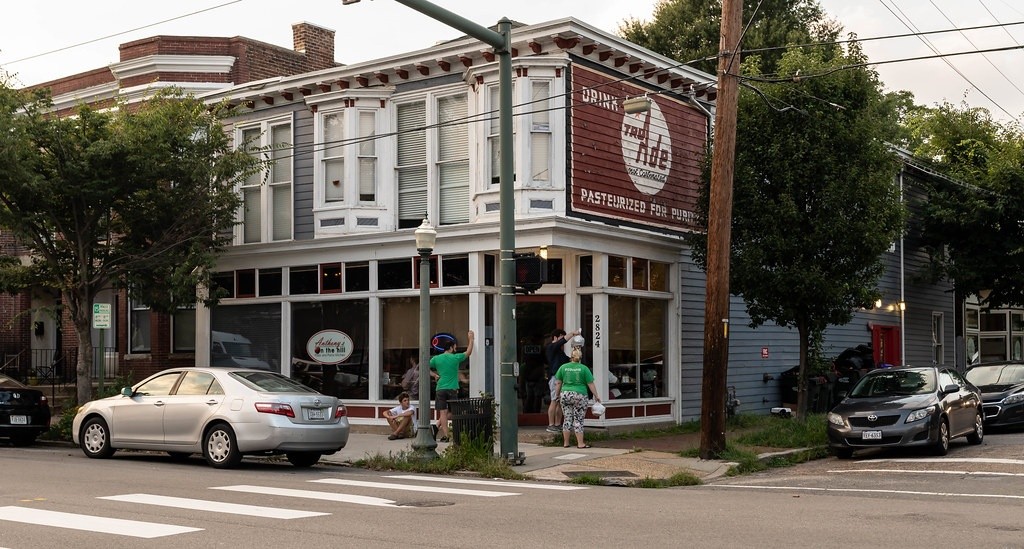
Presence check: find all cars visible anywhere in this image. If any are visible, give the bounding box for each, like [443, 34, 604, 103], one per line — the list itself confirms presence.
[211, 330, 468, 407]
[959, 359, 1024, 434]
[826, 362, 986, 460]
[0, 373, 52, 448]
[72, 366, 351, 469]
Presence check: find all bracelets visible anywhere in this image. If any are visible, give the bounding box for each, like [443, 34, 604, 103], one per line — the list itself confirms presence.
[573, 333, 574, 336]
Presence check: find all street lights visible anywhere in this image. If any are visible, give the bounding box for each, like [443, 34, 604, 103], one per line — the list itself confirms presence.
[407, 210, 441, 461]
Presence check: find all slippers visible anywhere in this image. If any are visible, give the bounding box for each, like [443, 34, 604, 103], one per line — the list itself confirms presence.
[577, 444, 591, 448]
[564, 445, 571, 448]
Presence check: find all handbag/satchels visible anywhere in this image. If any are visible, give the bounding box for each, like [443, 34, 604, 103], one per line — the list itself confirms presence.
[591, 401, 606, 417]
[571, 328, 584, 348]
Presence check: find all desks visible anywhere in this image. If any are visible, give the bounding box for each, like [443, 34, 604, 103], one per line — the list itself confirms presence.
[609, 380, 655, 399]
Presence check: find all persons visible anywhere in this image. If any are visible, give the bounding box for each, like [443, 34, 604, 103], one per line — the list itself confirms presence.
[541, 329, 581, 432]
[553, 350, 603, 449]
[523, 365, 549, 399]
[382, 393, 417, 440]
[395, 355, 420, 399]
[429, 331, 474, 442]
[609, 362, 644, 399]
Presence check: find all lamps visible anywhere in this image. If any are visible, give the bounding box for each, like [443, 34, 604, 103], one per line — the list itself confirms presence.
[896, 300, 906, 310]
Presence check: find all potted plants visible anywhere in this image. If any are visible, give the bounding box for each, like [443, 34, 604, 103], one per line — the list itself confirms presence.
[28, 369, 38, 386]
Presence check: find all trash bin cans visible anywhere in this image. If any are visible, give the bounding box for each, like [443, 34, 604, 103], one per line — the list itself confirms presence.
[446, 397, 495, 455]
[781, 367, 868, 415]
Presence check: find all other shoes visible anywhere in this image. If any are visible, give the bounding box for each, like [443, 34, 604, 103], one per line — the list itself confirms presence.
[388, 433, 398, 440]
[555, 424, 563, 432]
[397, 432, 404, 439]
[440, 436, 450, 443]
[546, 424, 559, 433]
[431, 424, 439, 441]
